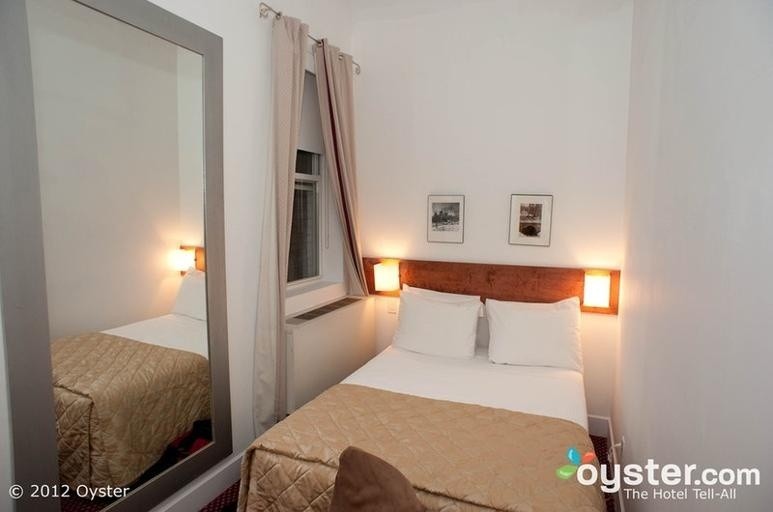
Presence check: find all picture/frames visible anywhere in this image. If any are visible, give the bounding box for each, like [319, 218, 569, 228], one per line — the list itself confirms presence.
[427, 194, 464, 245]
[506, 193, 553, 246]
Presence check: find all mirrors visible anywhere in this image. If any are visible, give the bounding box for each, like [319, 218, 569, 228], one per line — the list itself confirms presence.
[0, 1, 233, 510]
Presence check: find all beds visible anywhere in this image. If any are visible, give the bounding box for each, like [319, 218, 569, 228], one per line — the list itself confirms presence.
[237, 343, 609, 512]
[48, 313, 214, 498]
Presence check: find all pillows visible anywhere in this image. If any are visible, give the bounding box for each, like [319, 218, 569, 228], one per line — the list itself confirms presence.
[172, 269, 207, 320]
[393, 283, 583, 369]
